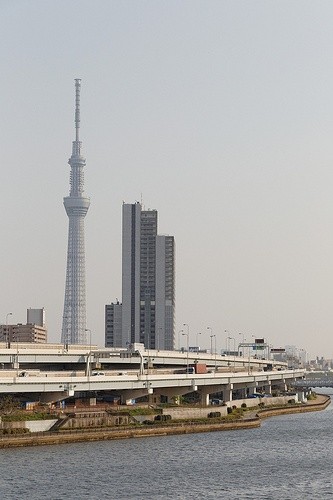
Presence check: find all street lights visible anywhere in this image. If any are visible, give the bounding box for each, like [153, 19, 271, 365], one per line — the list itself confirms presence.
[183, 333, 189, 351]
[229, 337, 236, 367]
[183, 323, 190, 373]
[141, 332, 150, 374]
[238, 332, 245, 366]
[85, 328, 91, 354]
[196, 333, 202, 353]
[6, 312, 12, 349]
[210, 336, 216, 371]
[207, 327, 213, 354]
